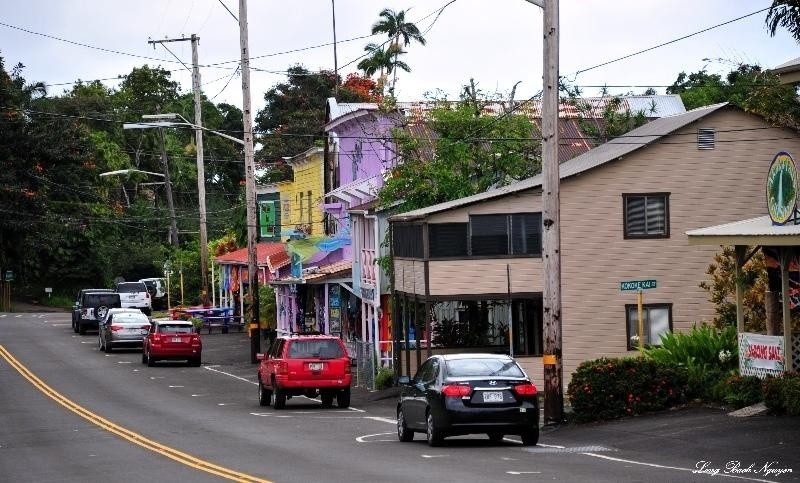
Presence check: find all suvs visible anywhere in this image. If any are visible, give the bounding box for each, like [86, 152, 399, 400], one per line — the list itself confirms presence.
[69, 273, 178, 353]
[252, 329, 355, 411]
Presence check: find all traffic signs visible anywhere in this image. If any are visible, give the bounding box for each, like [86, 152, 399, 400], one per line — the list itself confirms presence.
[620, 281, 658, 292]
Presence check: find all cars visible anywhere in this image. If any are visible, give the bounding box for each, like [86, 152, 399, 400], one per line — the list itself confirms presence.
[394, 350, 542, 447]
[140, 317, 202, 368]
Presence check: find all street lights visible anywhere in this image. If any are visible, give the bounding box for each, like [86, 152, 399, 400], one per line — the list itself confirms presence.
[119, 120, 264, 343]
[95, 168, 181, 249]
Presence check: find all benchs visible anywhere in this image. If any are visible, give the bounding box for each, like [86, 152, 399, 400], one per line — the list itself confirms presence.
[176, 303, 246, 335]
